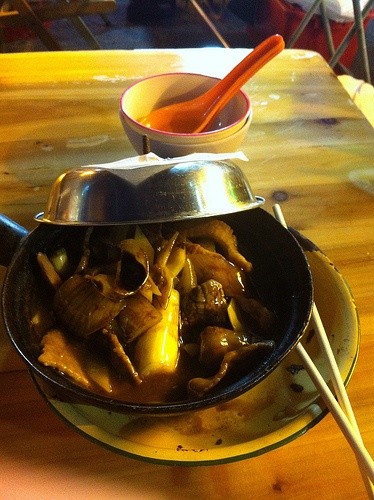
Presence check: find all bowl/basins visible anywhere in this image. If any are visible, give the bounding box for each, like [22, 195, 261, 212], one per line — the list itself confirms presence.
[34, 159, 266, 226]
[120, 73, 253, 160]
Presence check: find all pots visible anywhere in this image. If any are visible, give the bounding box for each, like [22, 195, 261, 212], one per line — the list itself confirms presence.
[0, 207, 313, 412]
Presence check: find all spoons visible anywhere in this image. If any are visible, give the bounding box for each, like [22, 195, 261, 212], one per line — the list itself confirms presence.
[147, 35, 284, 134]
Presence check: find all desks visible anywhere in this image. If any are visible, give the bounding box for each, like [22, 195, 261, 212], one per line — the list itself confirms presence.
[0, 48, 374, 500]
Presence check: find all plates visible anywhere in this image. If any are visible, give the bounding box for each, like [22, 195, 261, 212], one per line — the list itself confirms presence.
[29, 225, 361, 466]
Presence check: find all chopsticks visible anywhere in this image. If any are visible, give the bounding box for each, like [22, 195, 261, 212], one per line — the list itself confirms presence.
[272, 203, 374, 500]
